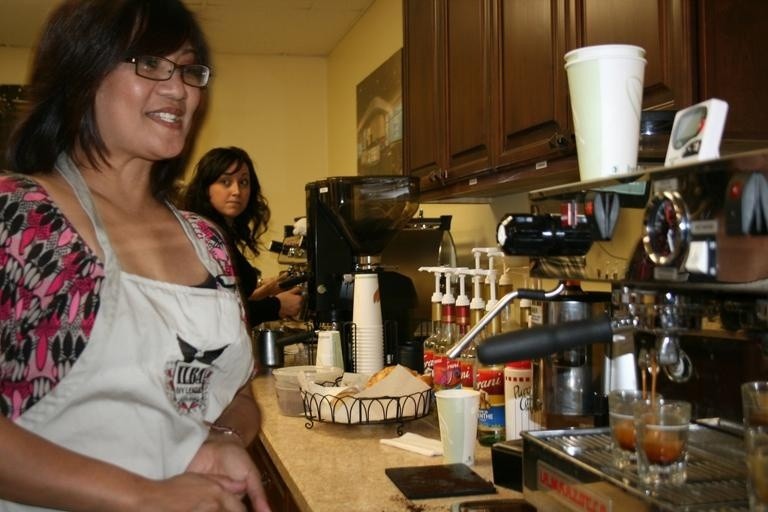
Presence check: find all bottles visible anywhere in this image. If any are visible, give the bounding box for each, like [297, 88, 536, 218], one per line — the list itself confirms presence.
[548, 275, 594, 430]
[416, 246, 542, 442]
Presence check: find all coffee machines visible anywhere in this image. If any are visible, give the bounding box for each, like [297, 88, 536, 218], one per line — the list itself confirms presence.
[316, 172, 418, 382]
[267, 214, 454, 344]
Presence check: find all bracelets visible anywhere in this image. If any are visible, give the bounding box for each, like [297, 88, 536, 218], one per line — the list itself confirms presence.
[203, 420, 244, 444]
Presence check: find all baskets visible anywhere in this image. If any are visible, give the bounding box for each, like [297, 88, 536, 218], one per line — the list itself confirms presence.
[300, 376, 433, 436]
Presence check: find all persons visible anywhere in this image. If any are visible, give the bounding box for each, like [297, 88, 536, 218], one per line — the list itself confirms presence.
[0, 0, 273, 512]
[179, 147, 304, 328]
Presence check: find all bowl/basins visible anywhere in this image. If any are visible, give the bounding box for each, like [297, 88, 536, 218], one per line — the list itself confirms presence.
[271, 364, 325, 417]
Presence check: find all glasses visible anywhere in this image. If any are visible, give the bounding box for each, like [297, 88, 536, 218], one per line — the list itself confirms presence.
[116, 49, 213, 89]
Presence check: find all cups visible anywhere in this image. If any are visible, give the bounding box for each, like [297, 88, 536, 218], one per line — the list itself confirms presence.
[352, 274, 386, 377]
[629, 396, 692, 485]
[562, 44, 645, 183]
[606, 387, 662, 469]
[435, 387, 482, 468]
[741, 379, 768, 512]
[314, 330, 345, 373]
[611, 287, 701, 330]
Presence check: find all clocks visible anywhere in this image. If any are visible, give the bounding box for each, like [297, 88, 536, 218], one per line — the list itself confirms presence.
[663, 97, 729, 168]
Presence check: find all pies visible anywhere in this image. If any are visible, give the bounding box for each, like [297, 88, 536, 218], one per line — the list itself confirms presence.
[329, 363, 435, 412]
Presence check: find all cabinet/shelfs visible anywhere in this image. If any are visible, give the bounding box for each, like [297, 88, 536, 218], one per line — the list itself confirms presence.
[402, 0, 767, 205]
